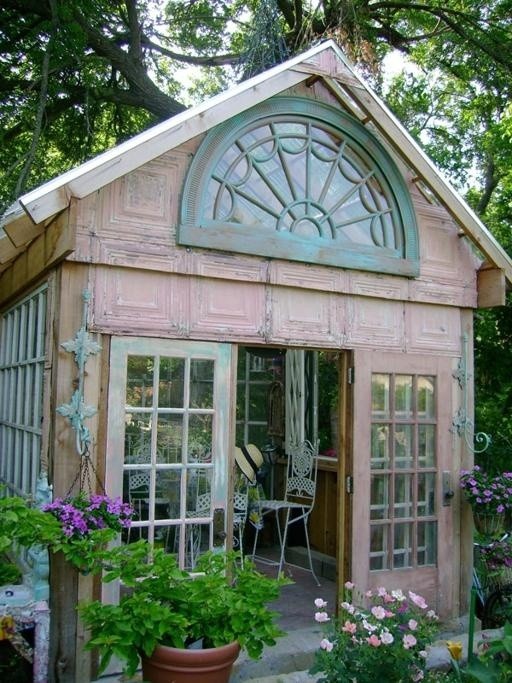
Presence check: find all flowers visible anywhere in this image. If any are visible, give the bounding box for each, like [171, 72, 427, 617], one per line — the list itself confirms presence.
[458, 465, 512, 513]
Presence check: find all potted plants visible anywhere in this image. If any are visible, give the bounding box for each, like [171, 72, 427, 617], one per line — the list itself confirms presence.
[75, 538, 296, 683]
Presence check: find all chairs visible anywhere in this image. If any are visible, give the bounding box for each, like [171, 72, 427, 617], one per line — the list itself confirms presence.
[128, 434, 322, 587]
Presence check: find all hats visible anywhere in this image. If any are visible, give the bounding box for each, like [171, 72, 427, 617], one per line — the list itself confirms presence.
[235, 444, 263, 484]
